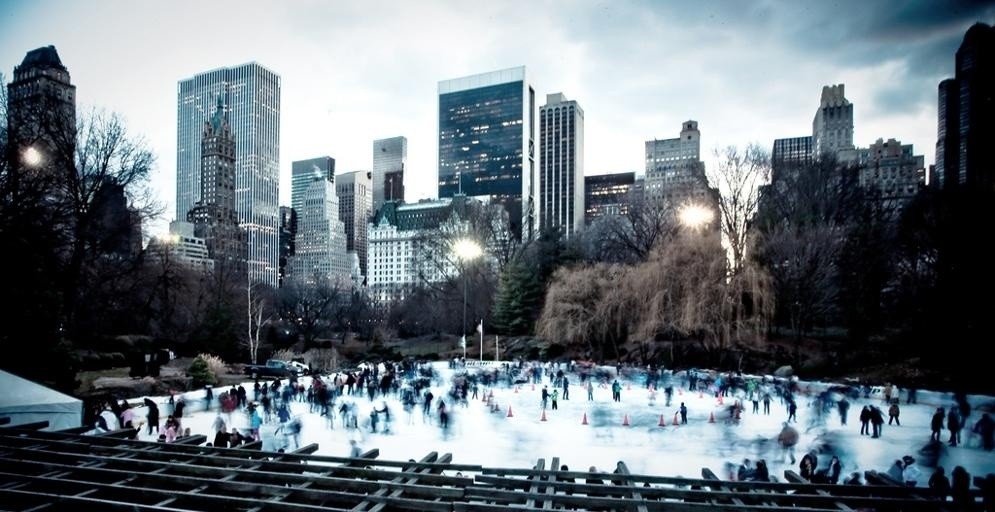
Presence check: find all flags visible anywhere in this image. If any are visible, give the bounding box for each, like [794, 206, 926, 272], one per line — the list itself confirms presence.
[458, 336, 466, 348]
[476, 324, 481, 332]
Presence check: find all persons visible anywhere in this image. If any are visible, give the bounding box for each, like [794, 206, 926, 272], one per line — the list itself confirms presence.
[718, 371, 995, 488]
[473, 347, 742, 427]
[101, 355, 474, 462]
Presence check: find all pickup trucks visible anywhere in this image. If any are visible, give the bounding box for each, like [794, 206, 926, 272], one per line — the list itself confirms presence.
[243, 359, 303, 380]
[286, 361, 309, 375]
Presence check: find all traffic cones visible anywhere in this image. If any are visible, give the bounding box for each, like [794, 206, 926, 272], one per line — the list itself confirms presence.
[482, 382, 536, 418]
[659, 414, 665, 426]
[698, 389, 743, 424]
[582, 412, 588, 424]
[672, 415, 679, 425]
[622, 414, 630, 425]
[540, 408, 547, 421]
[584, 378, 684, 395]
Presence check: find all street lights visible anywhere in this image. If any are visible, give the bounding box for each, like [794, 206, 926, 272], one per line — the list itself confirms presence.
[454, 240, 482, 362]
